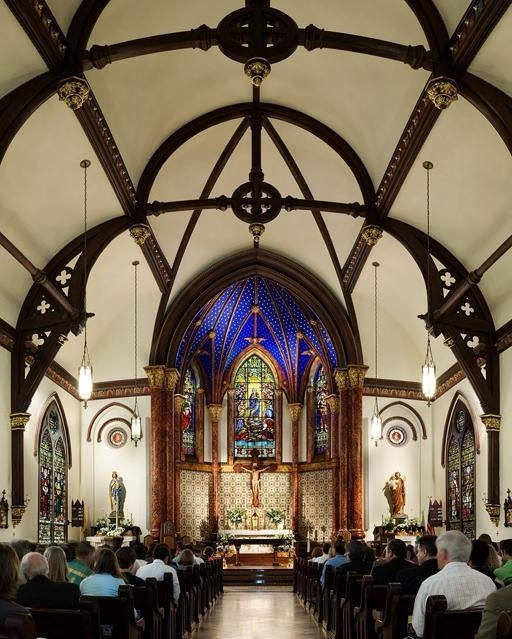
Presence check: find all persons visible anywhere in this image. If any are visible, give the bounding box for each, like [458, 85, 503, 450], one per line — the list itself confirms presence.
[110, 471, 122, 511]
[0, 541, 219, 639]
[388, 472, 406, 516]
[241, 465, 270, 499]
[389, 475, 400, 491]
[305, 528, 512, 639]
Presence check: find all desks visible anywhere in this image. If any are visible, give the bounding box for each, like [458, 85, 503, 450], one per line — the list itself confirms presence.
[229, 537, 290, 567]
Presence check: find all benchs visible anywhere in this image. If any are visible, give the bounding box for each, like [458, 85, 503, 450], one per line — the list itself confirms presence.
[1, 559, 223, 638]
[293, 555, 512, 638]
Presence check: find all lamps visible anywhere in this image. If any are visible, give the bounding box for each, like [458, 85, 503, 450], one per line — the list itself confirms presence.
[131, 260, 143, 448]
[77, 160, 93, 408]
[370, 262, 384, 448]
[422, 160, 437, 408]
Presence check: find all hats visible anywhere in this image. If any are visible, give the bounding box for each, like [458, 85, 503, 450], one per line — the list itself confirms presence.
[334, 544, 345, 554]
[347, 541, 364, 554]
